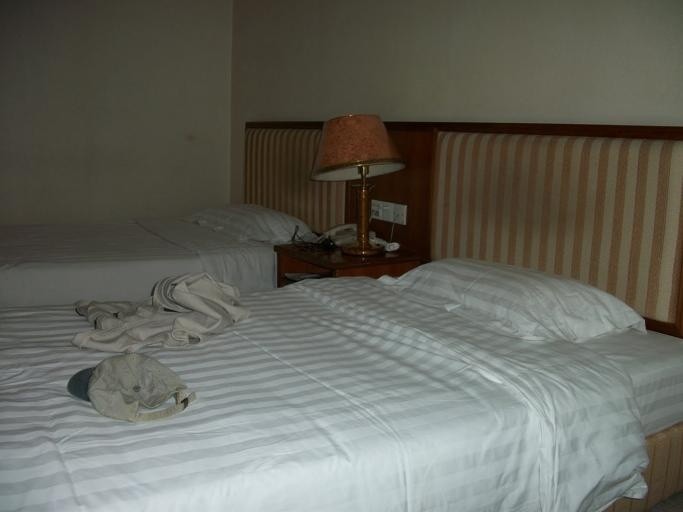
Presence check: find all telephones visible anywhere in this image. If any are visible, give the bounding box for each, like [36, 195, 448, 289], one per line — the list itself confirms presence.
[323, 222, 358, 247]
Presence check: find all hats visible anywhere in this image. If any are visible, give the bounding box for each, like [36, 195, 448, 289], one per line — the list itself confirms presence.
[65, 351, 198, 423]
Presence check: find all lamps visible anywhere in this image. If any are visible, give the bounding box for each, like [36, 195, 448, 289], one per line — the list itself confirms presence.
[309, 111, 407, 258]
